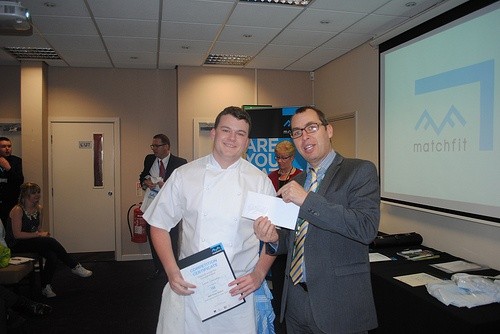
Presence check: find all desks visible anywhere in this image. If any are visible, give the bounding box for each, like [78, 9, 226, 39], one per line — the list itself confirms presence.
[367, 231, 500, 334]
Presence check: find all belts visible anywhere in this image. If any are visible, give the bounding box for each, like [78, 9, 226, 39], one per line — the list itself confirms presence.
[298, 282, 308, 292]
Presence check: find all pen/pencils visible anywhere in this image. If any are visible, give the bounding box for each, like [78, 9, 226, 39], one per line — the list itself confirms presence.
[11, 258, 21, 260]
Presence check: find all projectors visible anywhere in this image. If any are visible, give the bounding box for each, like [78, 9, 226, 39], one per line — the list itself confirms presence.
[0, 0, 32, 31]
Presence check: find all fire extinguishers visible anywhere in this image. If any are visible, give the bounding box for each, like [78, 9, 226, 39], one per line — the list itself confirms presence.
[127, 202, 147, 243]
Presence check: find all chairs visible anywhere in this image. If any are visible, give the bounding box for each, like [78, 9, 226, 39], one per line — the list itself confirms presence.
[13, 253, 44, 296]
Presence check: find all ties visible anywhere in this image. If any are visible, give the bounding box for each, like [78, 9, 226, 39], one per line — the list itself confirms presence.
[290, 167, 320, 286]
[159, 160, 165, 180]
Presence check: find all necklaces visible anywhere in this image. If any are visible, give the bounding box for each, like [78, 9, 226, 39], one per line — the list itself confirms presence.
[278, 166, 293, 190]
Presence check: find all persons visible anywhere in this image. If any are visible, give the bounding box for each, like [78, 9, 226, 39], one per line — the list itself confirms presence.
[0, 137, 24, 226]
[253, 106, 381, 334]
[5, 183, 93, 297]
[0, 285, 56, 334]
[142, 106, 277, 334]
[268, 140, 302, 197]
[140, 134, 188, 274]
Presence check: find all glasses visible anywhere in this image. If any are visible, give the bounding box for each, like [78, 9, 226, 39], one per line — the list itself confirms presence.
[150, 144, 165, 149]
[289, 122, 323, 139]
[275, 155, 291, 160]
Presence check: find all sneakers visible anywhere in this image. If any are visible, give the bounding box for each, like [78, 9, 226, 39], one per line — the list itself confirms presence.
[42, 284, 56, 298]
[72, 264, 92, 277]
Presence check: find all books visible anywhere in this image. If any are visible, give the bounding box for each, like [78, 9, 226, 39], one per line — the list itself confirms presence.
[397, 250, 434, 259]
